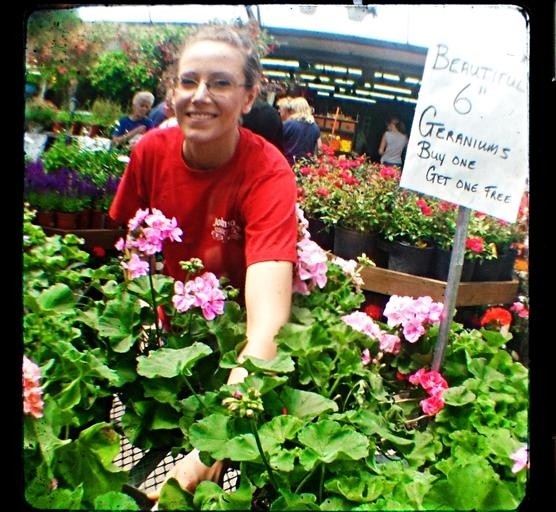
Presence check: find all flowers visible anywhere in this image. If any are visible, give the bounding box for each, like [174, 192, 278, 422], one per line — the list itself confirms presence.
[23, 119, 134, 210]
[292, 134, 529, 260]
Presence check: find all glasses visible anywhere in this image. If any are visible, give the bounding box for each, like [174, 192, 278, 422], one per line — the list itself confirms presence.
[170, 78, 254, 96]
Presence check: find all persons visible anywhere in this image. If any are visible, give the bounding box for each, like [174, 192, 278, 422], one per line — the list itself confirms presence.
[110, 25, 296, 509]
[112, 88, 322, 167]
[378, 118, 408, 168]
[27, 118, 100, 140]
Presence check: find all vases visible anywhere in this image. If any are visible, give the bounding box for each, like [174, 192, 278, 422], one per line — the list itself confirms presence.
[77, 209, 91, 228]
[333, 224, 379, 264]
[55, 211, 77, 229]
[379, 237, 436, 278]
[24, 205, 38, 224]
[431, 247, 478, 282]
[476, 253, 505, 281]
[106, 213, 122, 229]
[91, 210, 105, 228]
[306, 215, 332, 249]
[499, 248, 518, 281]
[37, 210, 54, 226]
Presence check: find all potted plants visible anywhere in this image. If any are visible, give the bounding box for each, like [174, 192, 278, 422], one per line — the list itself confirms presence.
[51, 110, 106, 138]
